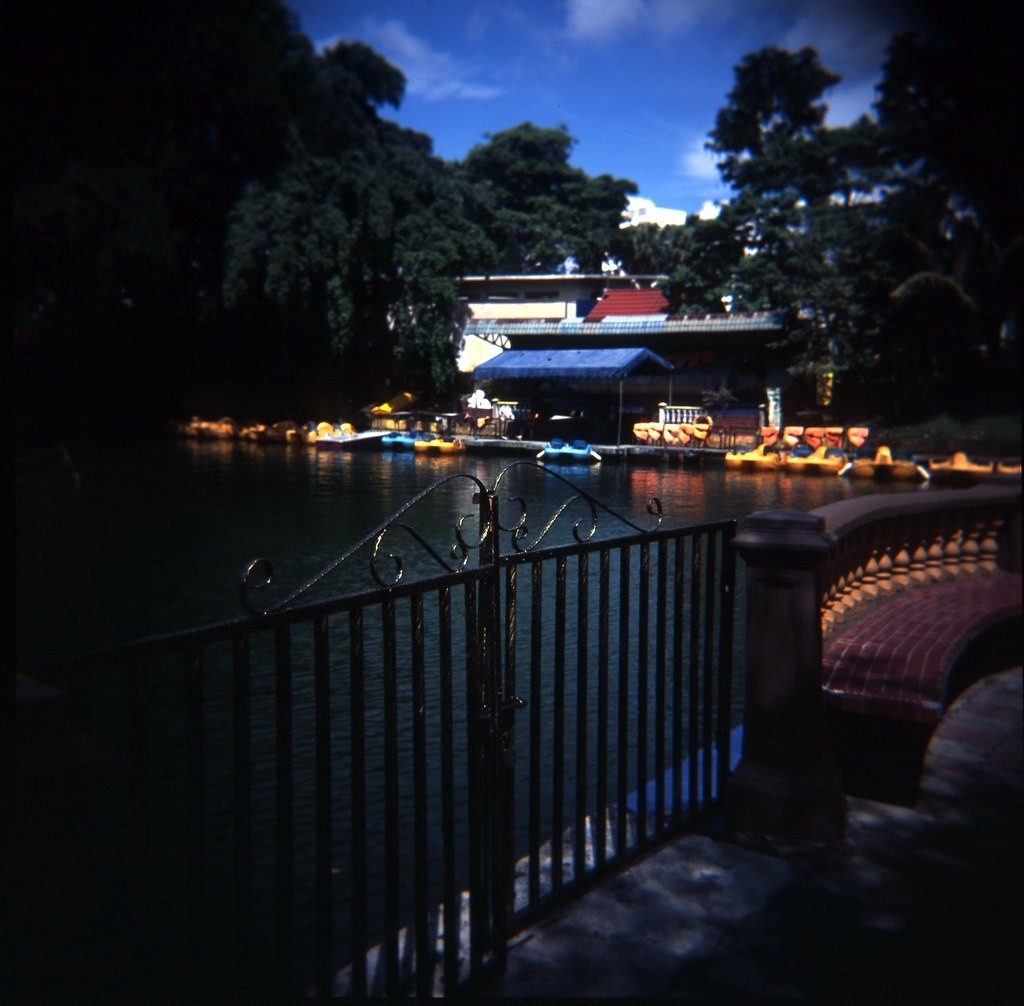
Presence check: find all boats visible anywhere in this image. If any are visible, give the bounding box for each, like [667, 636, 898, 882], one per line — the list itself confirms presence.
[542, 438, 596, 463]
[721, 442, 1021, 484]
[184, 415, 467, 454]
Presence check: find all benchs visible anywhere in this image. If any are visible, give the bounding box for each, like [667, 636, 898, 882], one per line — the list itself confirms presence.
[733, 486, 1024, 843]
